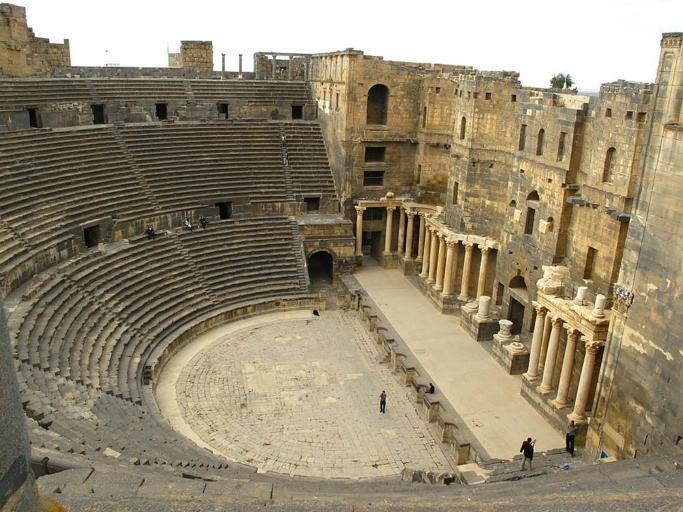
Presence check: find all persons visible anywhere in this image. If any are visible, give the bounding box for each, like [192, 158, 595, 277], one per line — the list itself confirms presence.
[564, 418, 577, 456]
[142, 226, 156, 240]
[520, 436, 537, 471]
[378, 391, 387, 414]
[199, 215, 209, 231]
[183, 218, 193, 232]
[424, 382, 436, 394]
